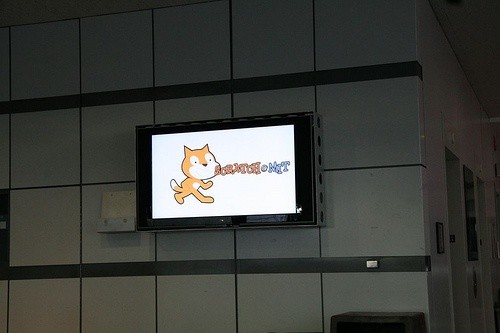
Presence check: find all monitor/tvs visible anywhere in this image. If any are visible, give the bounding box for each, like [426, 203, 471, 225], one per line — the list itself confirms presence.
[135, 112, 323, 233]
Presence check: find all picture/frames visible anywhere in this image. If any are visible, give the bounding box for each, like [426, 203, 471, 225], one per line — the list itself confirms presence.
[436, 221, 445, 254]
